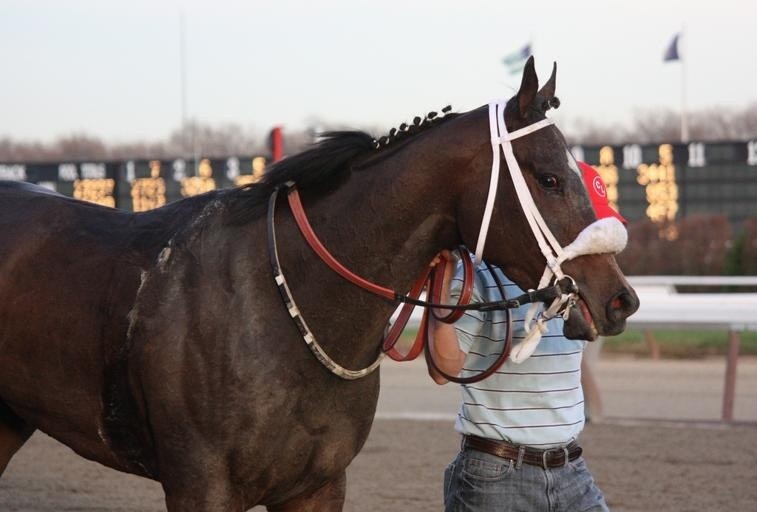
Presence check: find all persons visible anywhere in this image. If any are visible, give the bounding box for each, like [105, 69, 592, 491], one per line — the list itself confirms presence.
[423, 160, 629, 512]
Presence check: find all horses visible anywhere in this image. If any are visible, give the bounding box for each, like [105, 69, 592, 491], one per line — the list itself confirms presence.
[0, 54, 644, 512]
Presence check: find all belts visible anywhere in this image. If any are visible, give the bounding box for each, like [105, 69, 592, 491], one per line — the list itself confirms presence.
[464, 434, 583, 468]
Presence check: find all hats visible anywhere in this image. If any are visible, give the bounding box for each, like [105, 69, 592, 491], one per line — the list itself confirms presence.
[576, 161, 629, 226]
